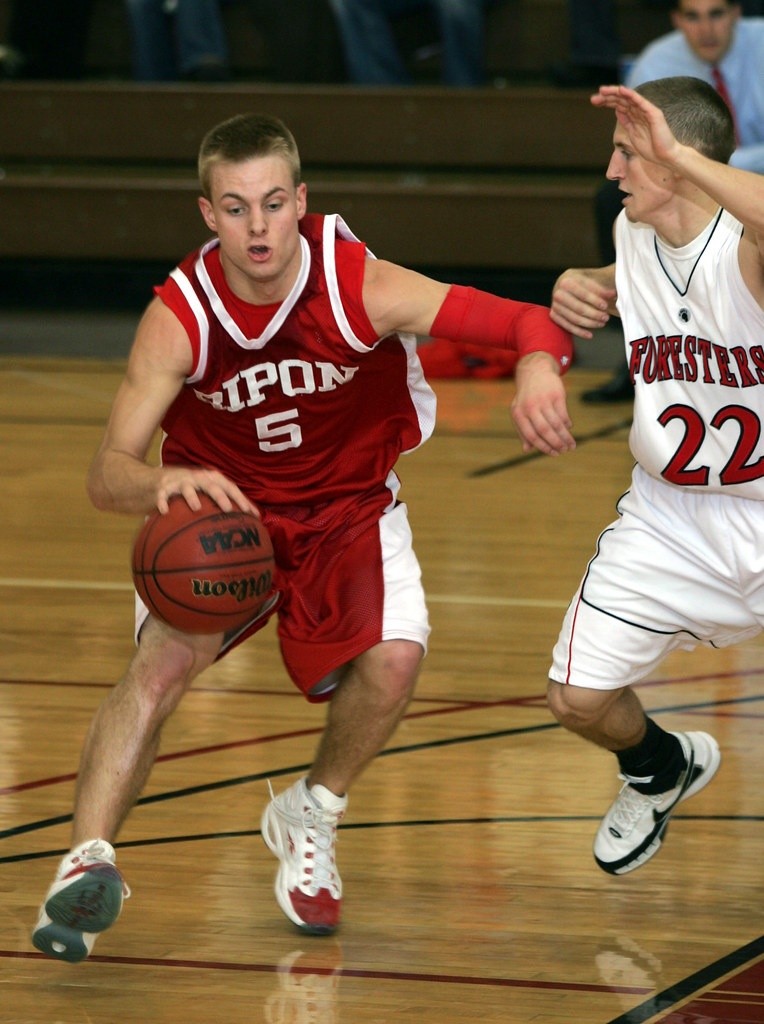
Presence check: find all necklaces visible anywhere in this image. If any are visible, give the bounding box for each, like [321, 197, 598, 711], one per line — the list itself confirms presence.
[653, 206, 726, 321]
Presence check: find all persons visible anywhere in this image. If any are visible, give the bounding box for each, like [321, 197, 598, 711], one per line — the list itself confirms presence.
[548, 74, 764, 876]
[537, 0, 764, 400]
[30, 117, 573, 960]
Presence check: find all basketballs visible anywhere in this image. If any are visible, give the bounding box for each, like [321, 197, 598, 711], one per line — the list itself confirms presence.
[129, 493, 275, 636]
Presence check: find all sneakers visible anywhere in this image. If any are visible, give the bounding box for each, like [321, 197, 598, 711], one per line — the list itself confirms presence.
[259, 776, 348, 936]
[31, 838, 130, 967]
[591, 729, 720, 878]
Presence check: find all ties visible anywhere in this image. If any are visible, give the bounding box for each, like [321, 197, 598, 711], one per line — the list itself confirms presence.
[711, 67, 739, 147]
